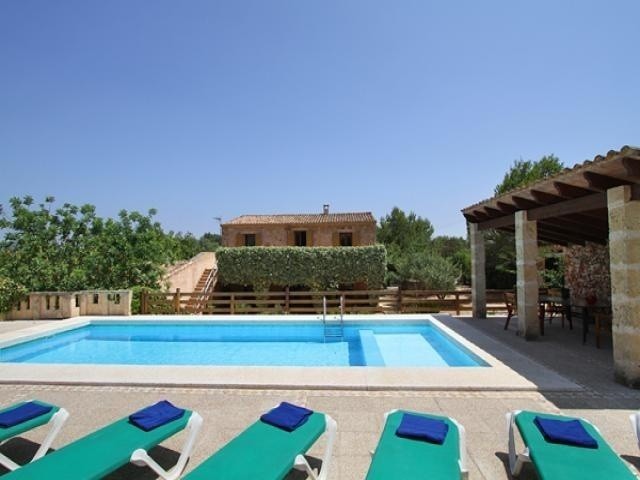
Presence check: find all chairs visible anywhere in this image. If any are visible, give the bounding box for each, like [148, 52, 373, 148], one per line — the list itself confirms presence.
[2, 400, 203, 480]
[508, 408, 638, 479]
[179, 400, 340, 479]
[1, 400, 69, 474]
[502, 286, 612, 348]
[365, 410, 466, 480]
